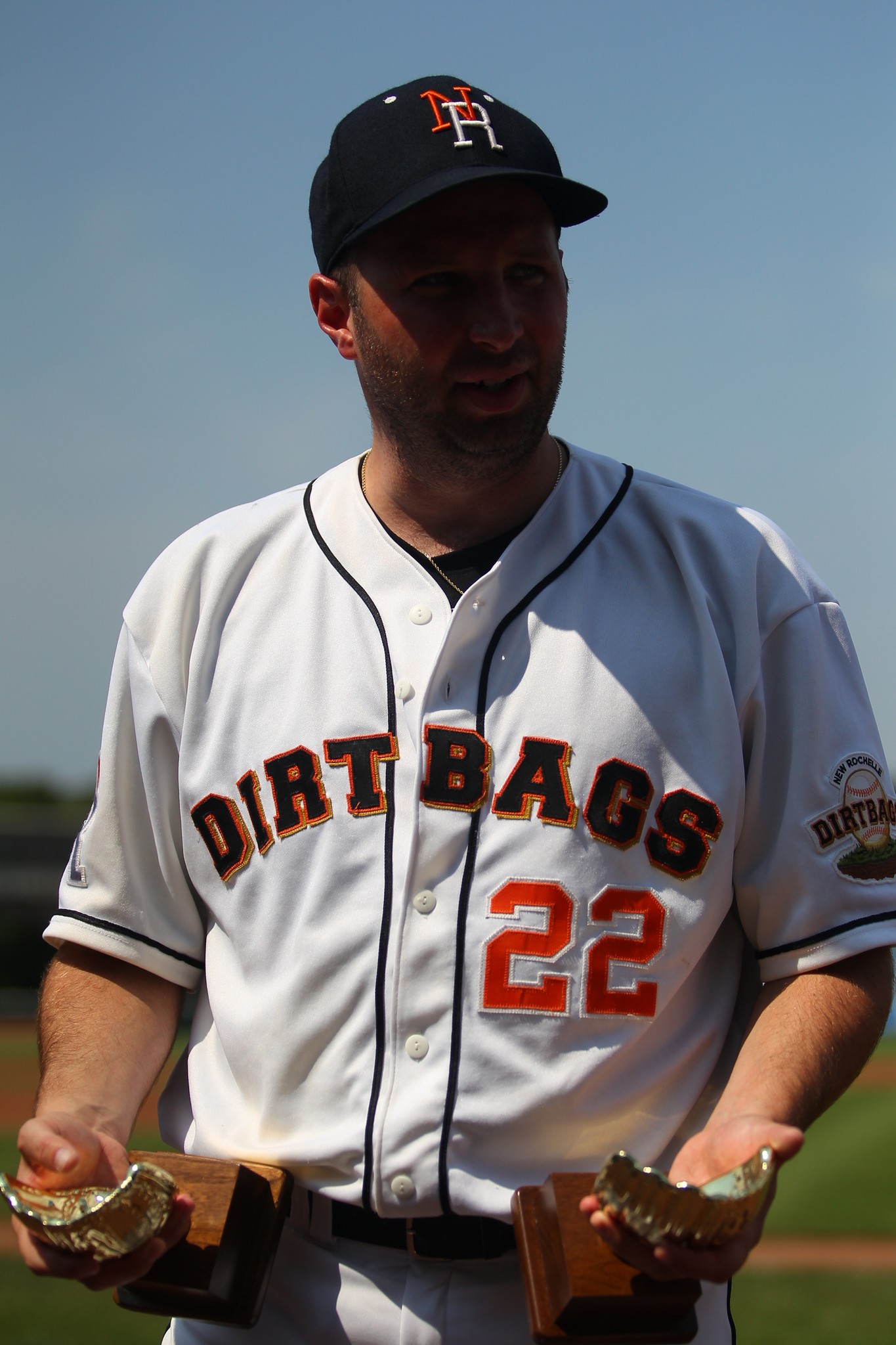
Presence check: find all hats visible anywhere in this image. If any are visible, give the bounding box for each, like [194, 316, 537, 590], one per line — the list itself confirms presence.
[309, 74, 609, 275]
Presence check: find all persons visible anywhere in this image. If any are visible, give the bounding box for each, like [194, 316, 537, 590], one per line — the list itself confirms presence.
[3, 75, 895, 1345]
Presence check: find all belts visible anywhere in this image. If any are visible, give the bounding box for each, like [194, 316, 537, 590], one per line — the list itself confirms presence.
[307, 1190, 518, 1260]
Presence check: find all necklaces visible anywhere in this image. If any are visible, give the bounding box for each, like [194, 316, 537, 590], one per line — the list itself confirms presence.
[361, 430, 563, 598]
